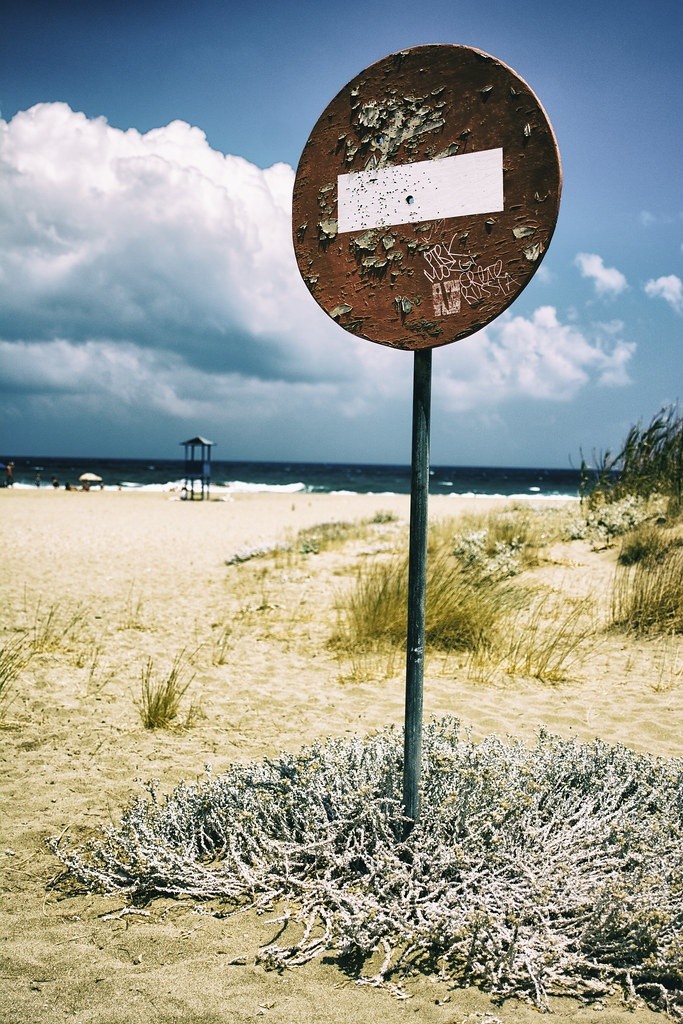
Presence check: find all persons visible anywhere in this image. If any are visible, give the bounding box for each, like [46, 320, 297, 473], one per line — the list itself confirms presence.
[51, 476, 59, 488]
[99, 481, 104, 489]
[82, 480, 91, 492]
[6, 461, 15, 487]
[36, 472, 41, 487]
[65, 482, 78, 491]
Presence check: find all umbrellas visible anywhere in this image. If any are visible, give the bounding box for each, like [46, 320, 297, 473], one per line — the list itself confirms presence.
[0, 463, 7, 469]
[79, 472, 102, 481]
